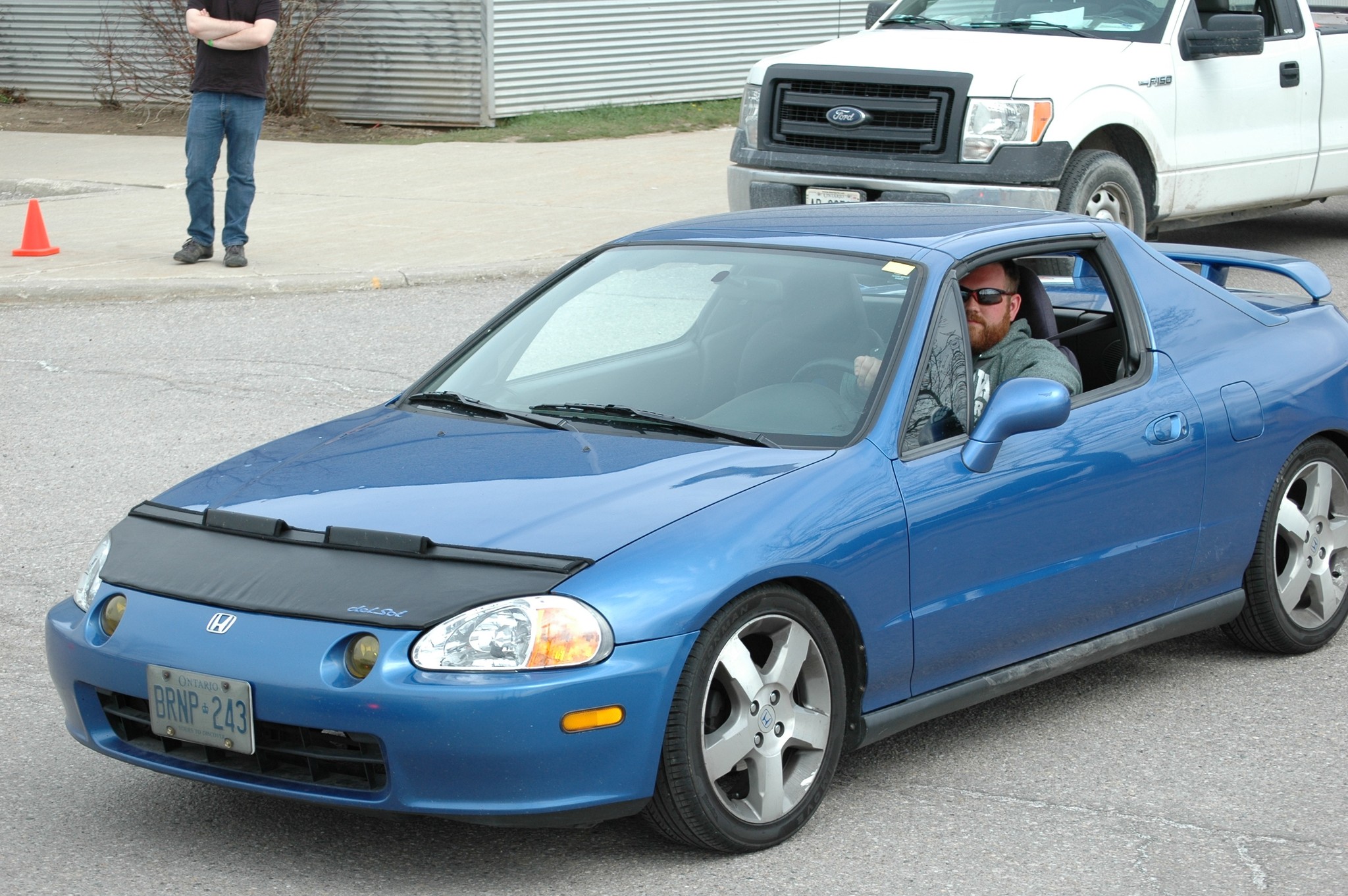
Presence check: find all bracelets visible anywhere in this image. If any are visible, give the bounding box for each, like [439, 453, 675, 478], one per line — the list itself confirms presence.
[209, 40, 213, 47]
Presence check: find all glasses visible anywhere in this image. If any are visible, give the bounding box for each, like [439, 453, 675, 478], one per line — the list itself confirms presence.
[959, 284, 1014, 305]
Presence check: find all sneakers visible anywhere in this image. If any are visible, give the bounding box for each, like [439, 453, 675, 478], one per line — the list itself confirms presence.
[173, 238, 214, 263]
[223, 244, 248, 267]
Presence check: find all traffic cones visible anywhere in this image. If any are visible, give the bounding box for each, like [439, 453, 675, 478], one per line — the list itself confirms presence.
[12, 199, 61, 257]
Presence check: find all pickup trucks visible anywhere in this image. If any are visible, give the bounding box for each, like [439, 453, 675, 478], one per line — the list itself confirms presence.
[724, 0, 1348, 266]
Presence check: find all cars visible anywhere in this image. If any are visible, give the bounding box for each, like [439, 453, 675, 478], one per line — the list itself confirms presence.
[43, 200, 1348, 860]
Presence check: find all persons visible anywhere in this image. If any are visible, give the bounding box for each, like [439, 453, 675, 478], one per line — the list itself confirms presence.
[173, 0, 281, 267]
[853, 261, 1082, 450]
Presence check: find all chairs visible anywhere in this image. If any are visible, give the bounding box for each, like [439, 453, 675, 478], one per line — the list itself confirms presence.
[1015, 264, 1082, 375]
[1196, 0, 1230, 30]
[1015, 0, 1101, 19]
[737, 261, 874, 404]
